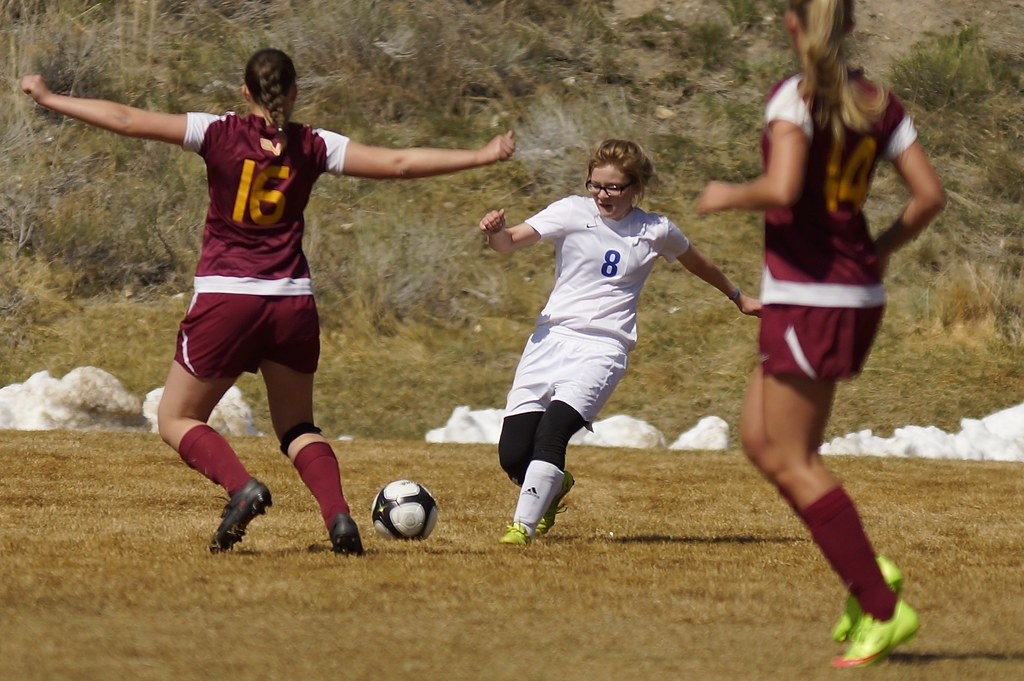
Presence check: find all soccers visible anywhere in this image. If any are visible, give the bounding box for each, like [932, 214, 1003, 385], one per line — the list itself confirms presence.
[371, 479, 440, 544]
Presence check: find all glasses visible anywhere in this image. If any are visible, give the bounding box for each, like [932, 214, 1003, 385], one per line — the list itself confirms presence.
[586, 179, 633, 198]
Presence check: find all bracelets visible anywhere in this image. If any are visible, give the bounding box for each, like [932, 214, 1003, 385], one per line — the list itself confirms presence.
[728, 288, 740, 301]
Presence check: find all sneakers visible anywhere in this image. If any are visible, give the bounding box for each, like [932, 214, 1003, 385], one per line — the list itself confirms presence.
[498, 524, 534, 545]
[208, 478, 272, 554]
[328, 512, 364, 555]
[535, 471, 574, 537]
[830, 554, 921, 668]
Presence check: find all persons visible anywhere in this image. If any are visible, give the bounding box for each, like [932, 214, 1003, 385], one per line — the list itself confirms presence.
[694, 1, 952, 671]
[21, 45, 517, 555]
[477, 138, 766, 550]
